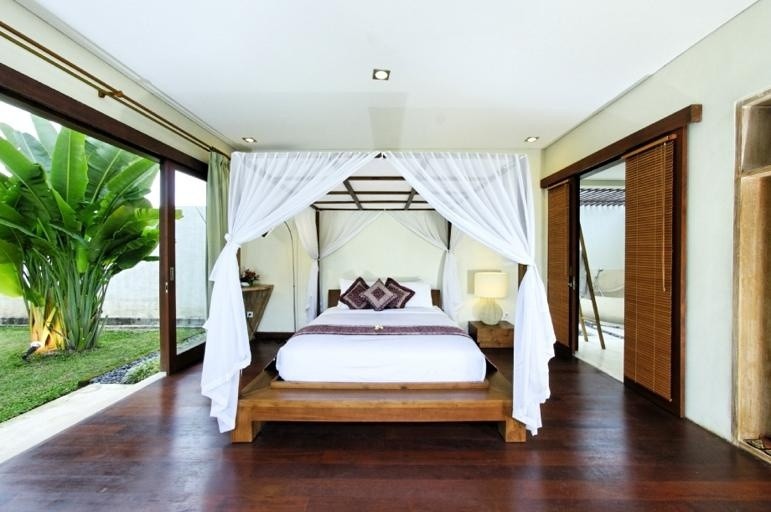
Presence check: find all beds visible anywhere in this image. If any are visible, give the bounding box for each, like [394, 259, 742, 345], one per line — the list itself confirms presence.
[228, 289, 529, 443]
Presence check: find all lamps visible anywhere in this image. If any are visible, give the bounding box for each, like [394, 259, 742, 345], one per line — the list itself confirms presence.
[474, 271, 508, 325]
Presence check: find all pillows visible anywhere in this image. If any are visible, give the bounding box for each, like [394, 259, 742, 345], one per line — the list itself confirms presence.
[338, 277, 433, 311]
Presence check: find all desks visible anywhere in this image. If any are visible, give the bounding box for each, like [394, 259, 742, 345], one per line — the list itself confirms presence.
[468, 321, 513, 348]
[240, 284, 274, 340]
